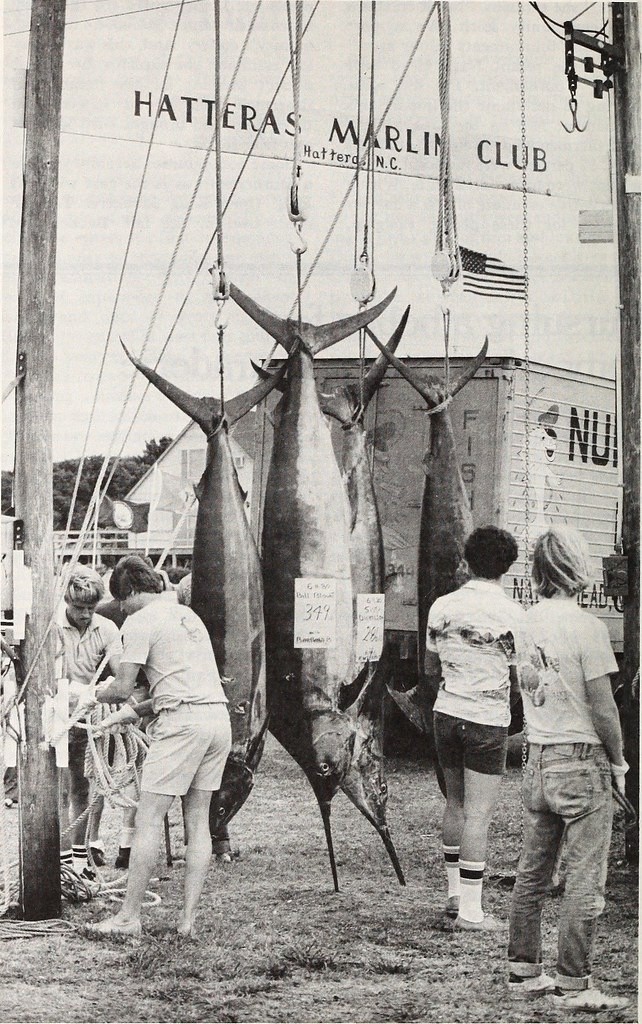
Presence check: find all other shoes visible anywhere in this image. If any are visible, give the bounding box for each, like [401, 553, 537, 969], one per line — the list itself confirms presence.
[176, 917, 196, 936]
[115, 845, 132, 868]
[446, 896, 463, 915]
[79, 868, 98, 882]
[453, 912, 510, 932]
[88, 848, 106, 865]
[85, 914, 143, 935]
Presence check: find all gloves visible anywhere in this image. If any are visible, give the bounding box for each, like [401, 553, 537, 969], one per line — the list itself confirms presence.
[100, 704, 140, 735]
[79, 686, 100, 710]
[611, 759, 630, 810]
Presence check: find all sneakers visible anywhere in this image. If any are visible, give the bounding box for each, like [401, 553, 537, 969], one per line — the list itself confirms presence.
[507, 972, 556, 992]
[552, 986, 630, 1009]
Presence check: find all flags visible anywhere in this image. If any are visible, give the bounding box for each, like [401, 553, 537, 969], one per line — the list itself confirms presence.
[459, 243, 528, 299]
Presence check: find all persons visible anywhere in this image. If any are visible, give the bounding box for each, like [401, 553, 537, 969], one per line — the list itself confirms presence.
[84, 553, 231, 939]
[59, 551, 193, 876]
[426, 525, 525, 932]
[508, 525, 630, 1009]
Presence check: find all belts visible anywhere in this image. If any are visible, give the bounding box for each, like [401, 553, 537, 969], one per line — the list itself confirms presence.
[160, 703, 225, 714]
